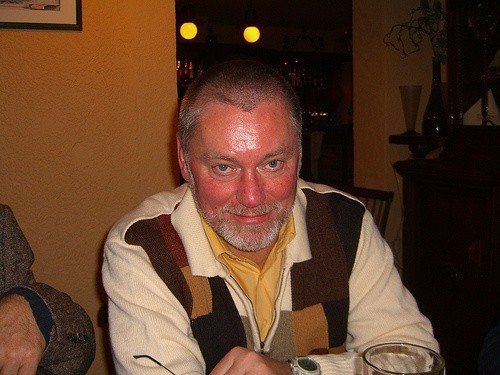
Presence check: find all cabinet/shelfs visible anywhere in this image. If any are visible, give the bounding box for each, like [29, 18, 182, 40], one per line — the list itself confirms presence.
[389, 127, 500, 375]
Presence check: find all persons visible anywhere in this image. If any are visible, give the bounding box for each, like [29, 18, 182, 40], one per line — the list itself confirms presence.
[0, 204, 97, 375]
[101, 60, 446, 375]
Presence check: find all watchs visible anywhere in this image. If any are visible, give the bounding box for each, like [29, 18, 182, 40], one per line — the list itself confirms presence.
[285, 355, 322, 375]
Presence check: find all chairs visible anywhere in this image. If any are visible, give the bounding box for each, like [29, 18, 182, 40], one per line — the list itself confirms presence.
[334, 188, 394, 238]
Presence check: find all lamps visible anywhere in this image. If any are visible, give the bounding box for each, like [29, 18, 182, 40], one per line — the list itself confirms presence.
[178, 45, 205, 88]
[238, 2, 265, 46]
[178, 1, 205, 43]
[276, 52, 314, 85]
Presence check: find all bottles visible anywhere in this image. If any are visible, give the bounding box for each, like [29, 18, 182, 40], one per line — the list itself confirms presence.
[276, 59, 334, 126]
[177, 60, 184, 84]
[184, 58, 190, 83]
[197, 64, 203, 77]
[422, 58, 448, 136]
[189, 58, 195, 83]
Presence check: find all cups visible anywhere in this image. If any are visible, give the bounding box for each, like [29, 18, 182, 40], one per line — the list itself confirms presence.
[400, 86, 422, 135]
[361, 342, 446, 375]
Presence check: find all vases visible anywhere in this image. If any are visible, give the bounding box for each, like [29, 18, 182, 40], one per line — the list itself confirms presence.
[397, 83, 422, 135]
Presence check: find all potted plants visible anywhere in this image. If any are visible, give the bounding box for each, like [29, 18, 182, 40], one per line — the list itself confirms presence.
[383, 0, 451, 138]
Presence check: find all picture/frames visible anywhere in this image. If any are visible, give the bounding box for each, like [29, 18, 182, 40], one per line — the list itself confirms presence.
[0, 0, 83, 30]
